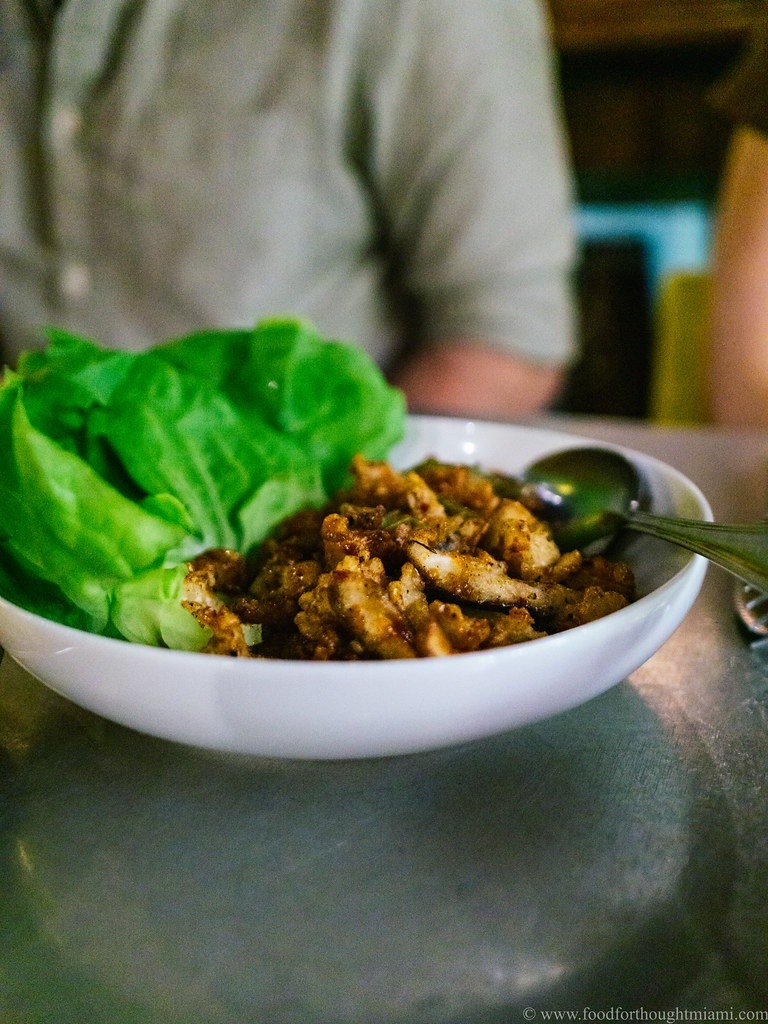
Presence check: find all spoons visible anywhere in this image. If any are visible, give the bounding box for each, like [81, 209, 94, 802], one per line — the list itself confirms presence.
[525, 447, 768, 592]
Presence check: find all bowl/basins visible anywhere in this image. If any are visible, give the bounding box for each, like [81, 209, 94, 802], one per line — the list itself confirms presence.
[1, 416, 716, 761]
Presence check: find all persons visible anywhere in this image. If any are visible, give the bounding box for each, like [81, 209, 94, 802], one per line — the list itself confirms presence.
[705, 41, 768, 434]
[0, 0, 579, 415]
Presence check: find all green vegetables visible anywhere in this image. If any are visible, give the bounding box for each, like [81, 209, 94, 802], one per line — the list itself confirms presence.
[0, 316, 410, 653]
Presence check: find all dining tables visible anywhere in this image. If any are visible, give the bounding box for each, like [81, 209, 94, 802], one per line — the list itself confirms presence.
[0, 417, 768, 1024]
